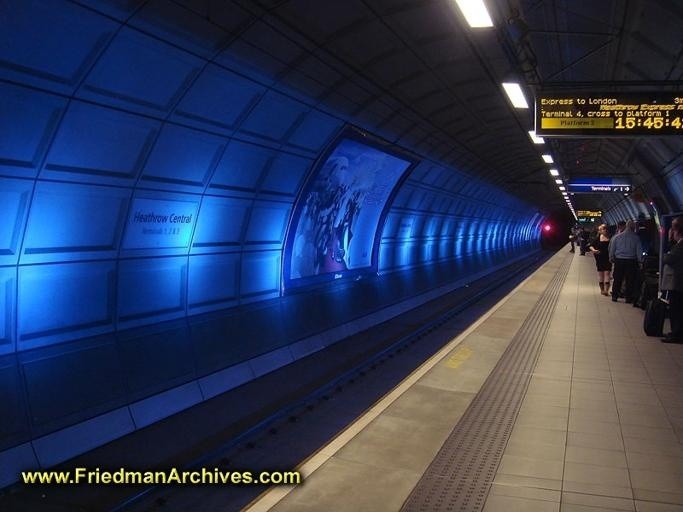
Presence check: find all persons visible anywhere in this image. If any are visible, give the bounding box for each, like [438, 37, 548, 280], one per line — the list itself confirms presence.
[569, 218, 644, 304]
[660, 217, 683, 344]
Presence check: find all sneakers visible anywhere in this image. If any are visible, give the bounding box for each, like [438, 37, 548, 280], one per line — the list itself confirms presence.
[661, 335, 683, 344]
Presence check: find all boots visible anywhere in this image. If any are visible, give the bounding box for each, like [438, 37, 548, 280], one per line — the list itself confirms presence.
[599, 282, 610, 296]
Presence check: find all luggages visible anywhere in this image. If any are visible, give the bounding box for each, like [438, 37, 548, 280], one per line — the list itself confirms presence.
[643, 289, 669, 337]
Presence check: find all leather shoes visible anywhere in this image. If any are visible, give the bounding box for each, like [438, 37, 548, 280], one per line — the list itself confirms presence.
[610, 292, 631, 303]
